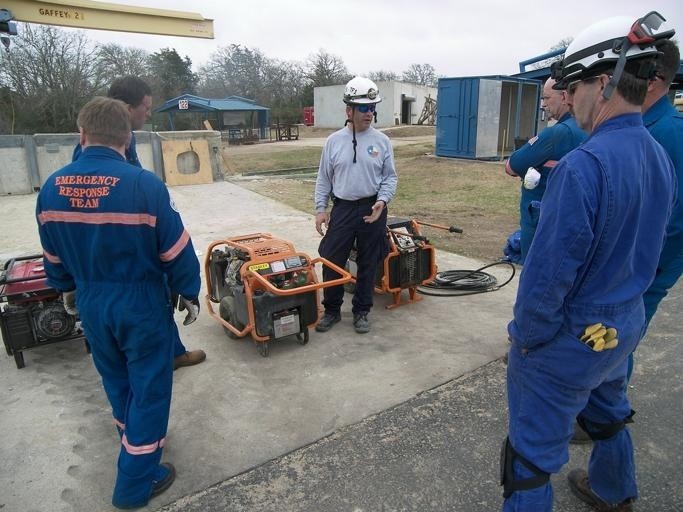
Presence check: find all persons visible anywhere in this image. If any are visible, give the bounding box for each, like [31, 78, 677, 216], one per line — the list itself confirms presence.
[71, 74, 206, 375]
[312, 74, 398, 337]
[500, 74, 590, 268]
[563, 34, 683, 447]
[497, 7, 679, 512]
[34, 93, 203, 512]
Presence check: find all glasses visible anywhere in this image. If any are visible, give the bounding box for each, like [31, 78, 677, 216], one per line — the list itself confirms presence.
[629, 11, 675, 44]
[352, 103, 376, 111]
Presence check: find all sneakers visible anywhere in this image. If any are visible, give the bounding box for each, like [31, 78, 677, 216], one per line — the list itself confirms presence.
[151, 463, 176, 495]
[174, 350, 206, 370]
[354, 310, 369, 332]
[316, 313, 341, 331]
[568, 468, 634, 511]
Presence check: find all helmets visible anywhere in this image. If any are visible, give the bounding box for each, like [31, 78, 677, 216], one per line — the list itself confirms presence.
[549, 10, 664, 90]
[343, 77, 381, 105]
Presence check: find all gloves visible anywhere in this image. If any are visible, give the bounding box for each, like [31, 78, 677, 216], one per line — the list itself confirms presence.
[179, 296, 200, 325]
[63, 288, 78, 316]
